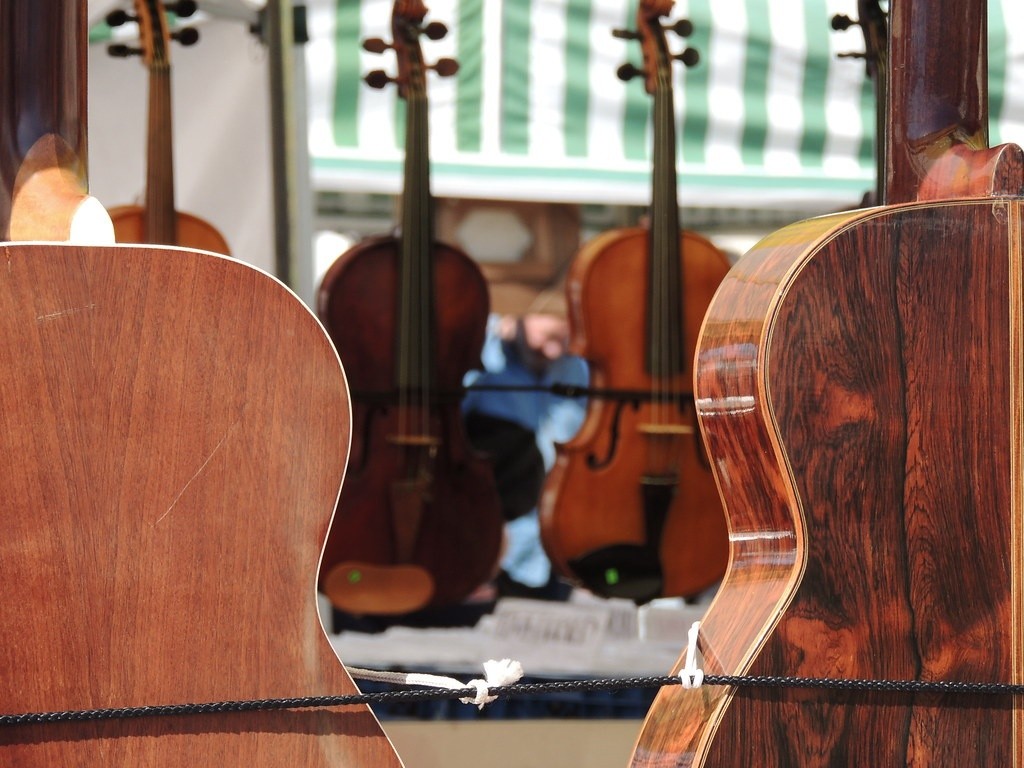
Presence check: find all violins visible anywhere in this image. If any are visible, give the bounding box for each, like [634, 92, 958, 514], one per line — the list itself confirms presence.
[3, 242, 406, 767]
[542, 0, 733, 603]
[108, 0, 227, 248]
[320, 0, 504, 620]
[627, 1, 1020, 768]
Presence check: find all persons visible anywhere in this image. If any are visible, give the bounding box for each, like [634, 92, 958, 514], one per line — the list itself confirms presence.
[466, 312, 594, 601]
